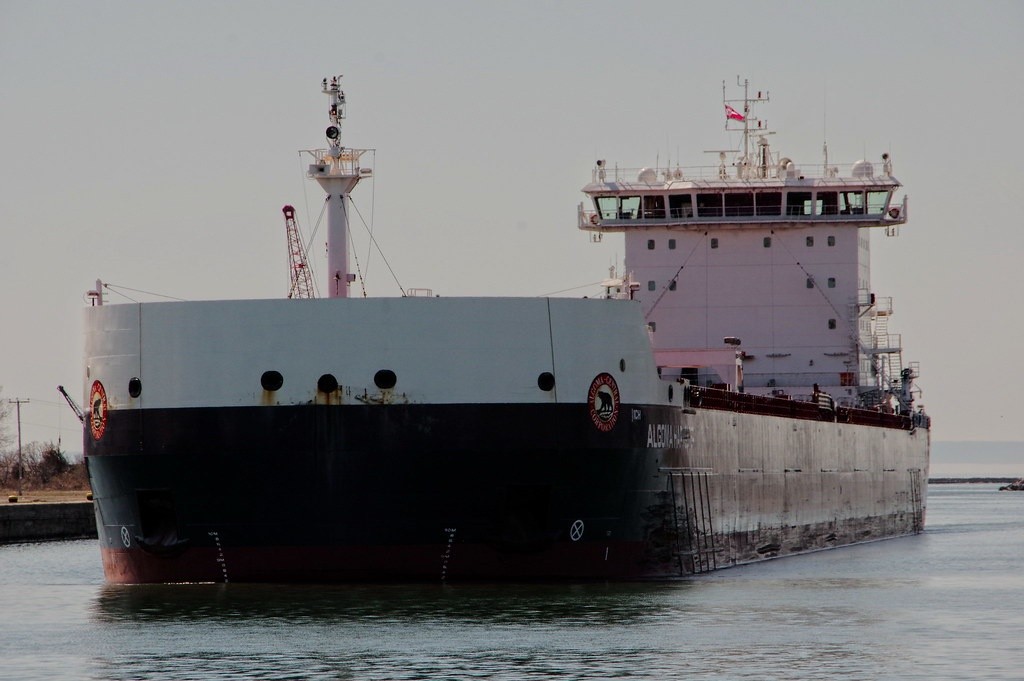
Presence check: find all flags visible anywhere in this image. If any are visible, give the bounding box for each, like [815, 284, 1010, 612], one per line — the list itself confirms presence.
[725, 104, 745, 121]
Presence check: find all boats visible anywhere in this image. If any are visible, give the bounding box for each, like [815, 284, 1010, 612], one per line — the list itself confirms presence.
[81, 72, 933, 590]
[998, 478, 1024, 491]
